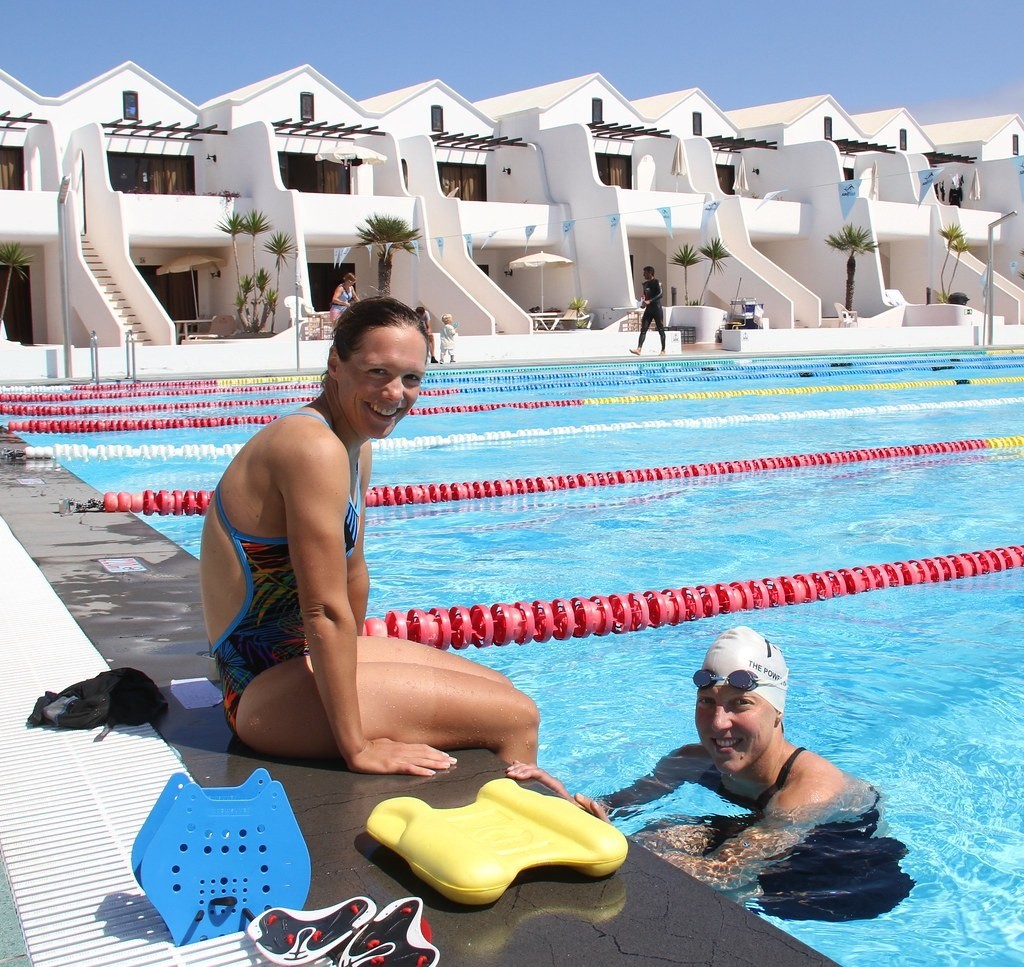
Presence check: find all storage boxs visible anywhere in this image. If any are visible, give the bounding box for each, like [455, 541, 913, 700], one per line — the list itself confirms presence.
[736, 304, 763, 329]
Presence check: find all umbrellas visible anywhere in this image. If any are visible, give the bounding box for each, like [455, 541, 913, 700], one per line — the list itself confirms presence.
[969, 168, 981, 210]
[671, 138, 687, 193]
[870, 160, 878, 200]
[732, 155, 749, 196]
[156, 251, 228, 333]
[315, 146, 387, 195]
[508, 251, 574, 326]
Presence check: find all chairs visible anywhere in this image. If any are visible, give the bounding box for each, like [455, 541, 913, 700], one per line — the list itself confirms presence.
[283, 295, 316, 340]
[834, 301, 859, 328]
[210, 314, 236, 340]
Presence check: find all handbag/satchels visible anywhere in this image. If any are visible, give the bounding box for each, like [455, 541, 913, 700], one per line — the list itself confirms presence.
[26, 667, 170, 743]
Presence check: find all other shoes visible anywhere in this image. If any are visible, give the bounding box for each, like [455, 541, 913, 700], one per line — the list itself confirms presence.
[659, 351, 665, 356]
[629, 348, 642, 355]
[431, 357, 438, 363]
[450, 360, 456, 362]
[440, 360, 444, 364]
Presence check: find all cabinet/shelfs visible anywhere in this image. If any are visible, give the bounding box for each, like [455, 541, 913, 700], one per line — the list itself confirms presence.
[718, 297, 758, 338]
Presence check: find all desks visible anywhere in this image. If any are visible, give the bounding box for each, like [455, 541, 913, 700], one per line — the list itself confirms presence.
[173, 318, 213, 344]
[612, 305, 659, 332]
[306, 311, 332, 340]
[526, 311, 565, 332]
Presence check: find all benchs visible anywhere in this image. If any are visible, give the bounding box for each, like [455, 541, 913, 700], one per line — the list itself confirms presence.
[530, 311, 595, 332]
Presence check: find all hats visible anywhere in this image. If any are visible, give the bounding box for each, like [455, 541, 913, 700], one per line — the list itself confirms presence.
[703, 626, 788, 715]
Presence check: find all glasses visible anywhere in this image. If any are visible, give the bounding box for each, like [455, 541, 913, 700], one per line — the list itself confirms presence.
[348, 280, 354, 285]
[693, 669, 760, 691]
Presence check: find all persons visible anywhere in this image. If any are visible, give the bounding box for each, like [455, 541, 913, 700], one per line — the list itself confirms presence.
[440, 314, 458, 364]
[411, 307, 438, 363]
[200, 296, 541, 778]
[330, 273, 361, 330]
[629, 266, 666, 356]
[507, 626, 880, 892]
